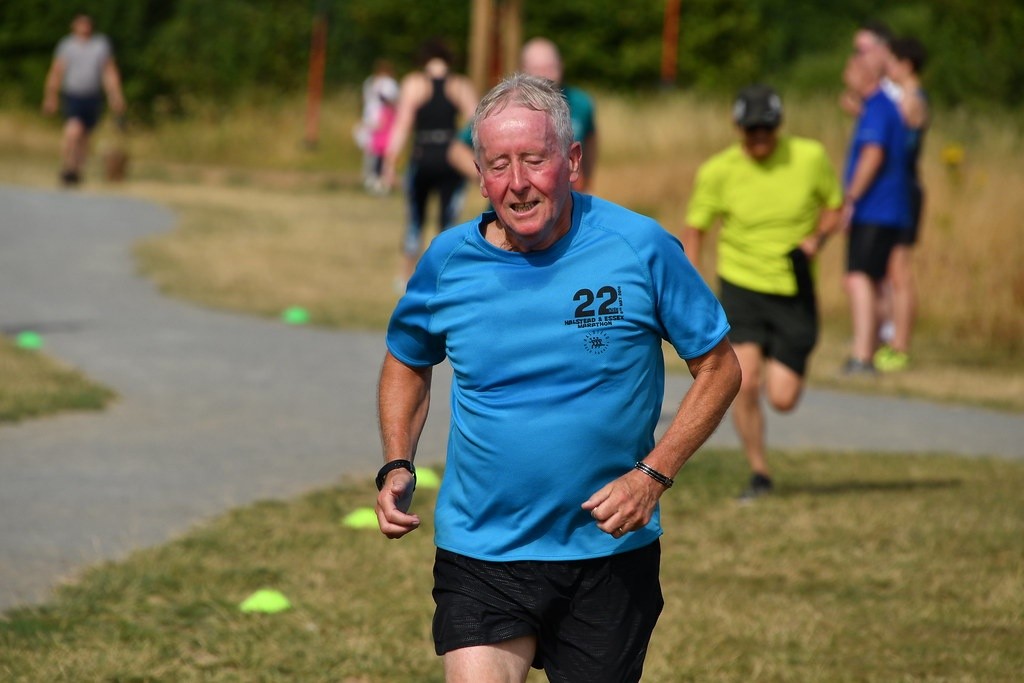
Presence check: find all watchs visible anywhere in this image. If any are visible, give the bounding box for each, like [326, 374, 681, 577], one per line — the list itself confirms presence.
[375, 460, 418, 494]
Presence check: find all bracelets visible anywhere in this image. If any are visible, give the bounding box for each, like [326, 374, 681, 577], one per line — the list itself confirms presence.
[635, 461, 674, 489]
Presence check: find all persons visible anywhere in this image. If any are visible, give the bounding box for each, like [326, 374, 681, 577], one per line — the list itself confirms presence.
[43, 10, 126, 185]
[374, 74, 741, 683]
[449, 38, 600, 195]
[381, 39, 480, 288]
[362, 58, 399, 195]
[833, 22, 930, 379]
[684, 88, 843, 505]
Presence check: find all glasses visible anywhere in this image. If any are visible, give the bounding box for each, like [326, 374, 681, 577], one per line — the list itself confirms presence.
[743, 126, 773, 134]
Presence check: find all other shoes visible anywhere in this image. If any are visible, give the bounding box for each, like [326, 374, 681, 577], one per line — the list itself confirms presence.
[748, 473, 769, 494]
[872, 345, 912, 372]
[844, 358, 870, 377]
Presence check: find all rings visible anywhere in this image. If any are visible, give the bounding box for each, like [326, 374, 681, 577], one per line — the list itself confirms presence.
[617, 528, 626, 536]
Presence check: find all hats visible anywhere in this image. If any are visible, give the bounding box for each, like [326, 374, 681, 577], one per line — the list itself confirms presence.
[736, 87, 782, 129]
